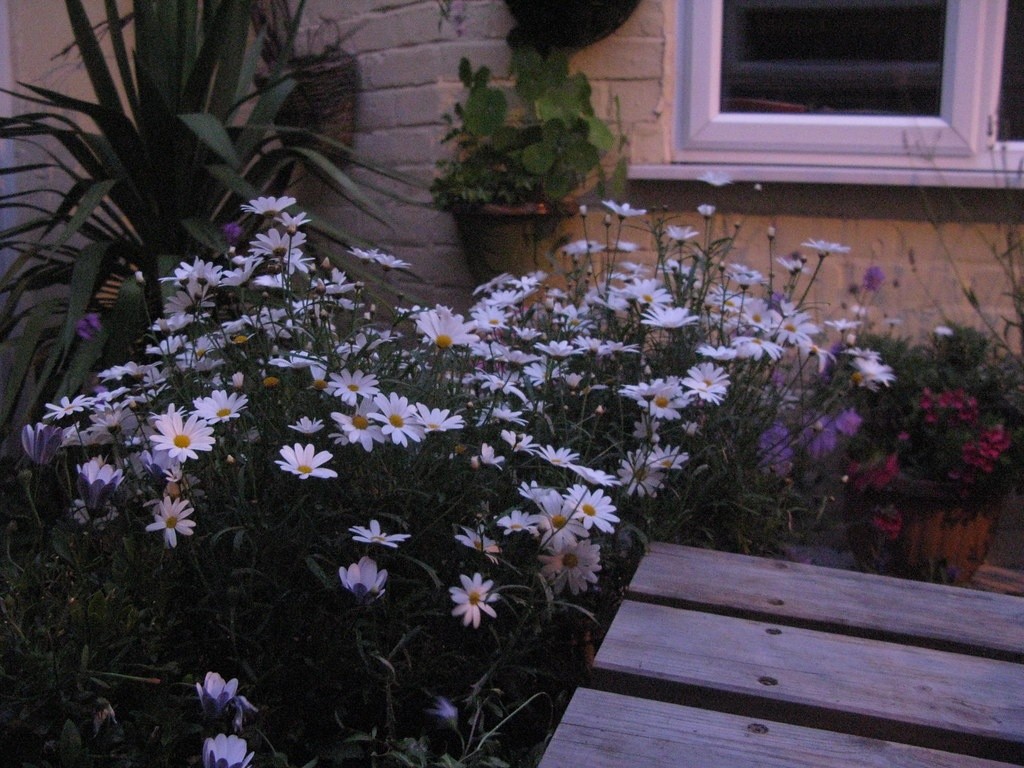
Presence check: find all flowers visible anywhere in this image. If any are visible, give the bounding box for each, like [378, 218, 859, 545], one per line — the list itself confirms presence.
[841, 320, 1024, 537]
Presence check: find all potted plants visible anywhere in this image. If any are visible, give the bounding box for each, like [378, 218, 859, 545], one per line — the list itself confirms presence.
[249, 0, 359, 164]
[430, 40, 634, 289]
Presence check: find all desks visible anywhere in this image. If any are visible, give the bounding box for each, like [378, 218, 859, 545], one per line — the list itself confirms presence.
[536, 537, 1024, 768]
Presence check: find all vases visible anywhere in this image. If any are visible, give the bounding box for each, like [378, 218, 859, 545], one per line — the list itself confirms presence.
[857, 488, 1002, 587]
